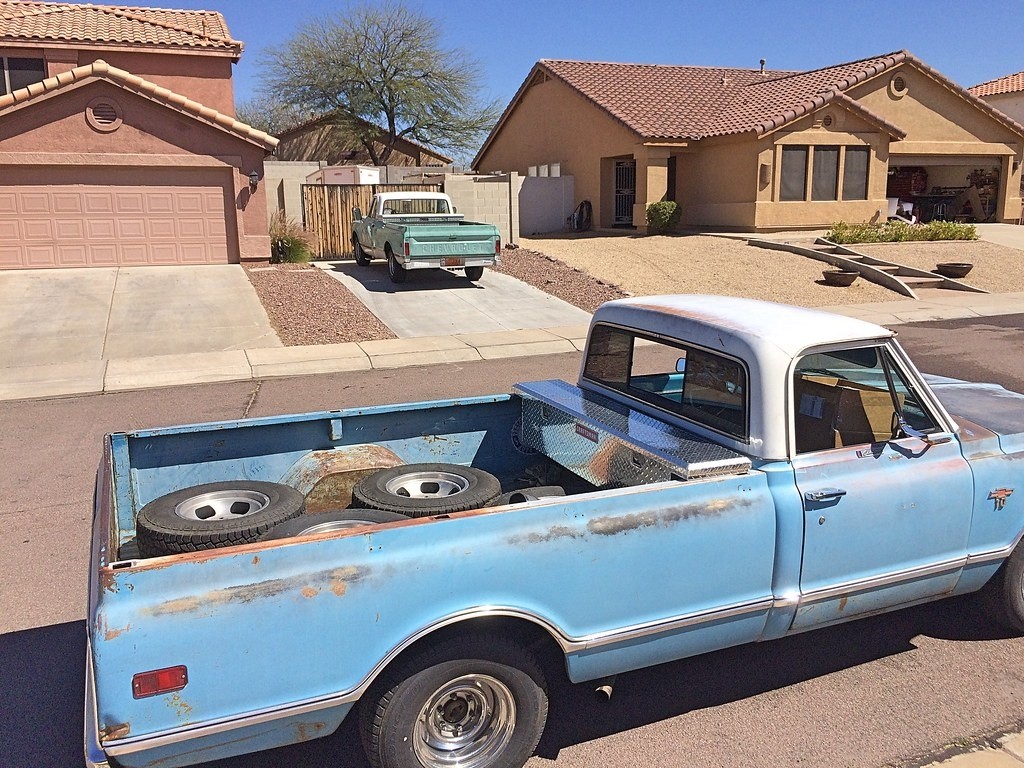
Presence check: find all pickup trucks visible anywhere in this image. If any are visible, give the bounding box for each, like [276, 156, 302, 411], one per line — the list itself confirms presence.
[83, 294, 1023, 768]
[351, 191, 501, 285]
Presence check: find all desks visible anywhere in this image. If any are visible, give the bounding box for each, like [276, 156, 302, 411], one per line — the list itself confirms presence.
[910, 193, 957, 220]
[979, 195, 997, 217]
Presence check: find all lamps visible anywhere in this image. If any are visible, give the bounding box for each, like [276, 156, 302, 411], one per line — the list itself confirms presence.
[249, 170, 259, 189]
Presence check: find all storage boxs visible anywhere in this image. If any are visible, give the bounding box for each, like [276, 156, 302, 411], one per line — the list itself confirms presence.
[794, 374, 905, 453]
